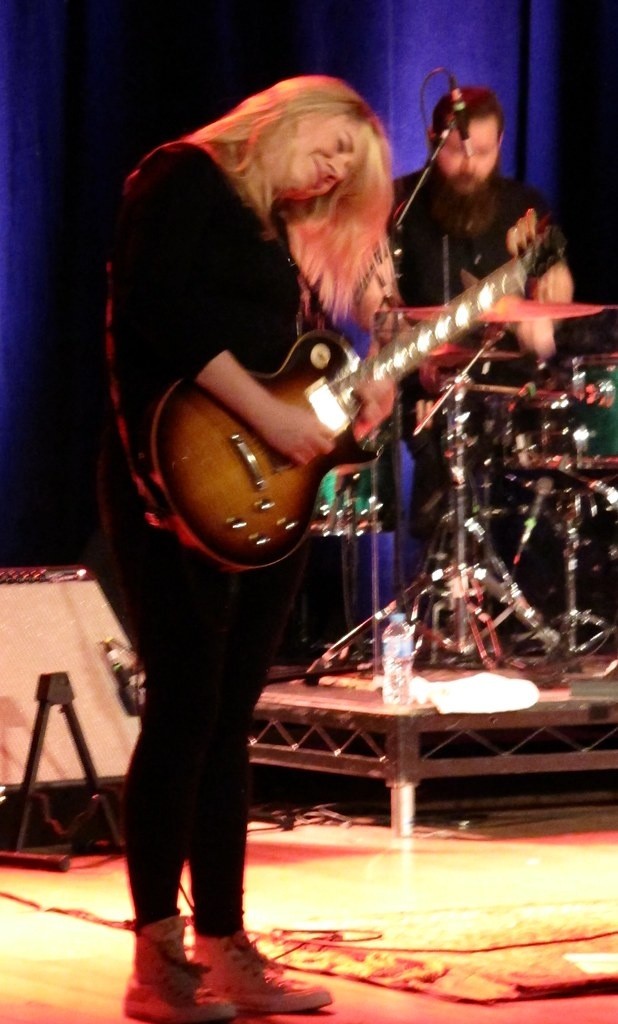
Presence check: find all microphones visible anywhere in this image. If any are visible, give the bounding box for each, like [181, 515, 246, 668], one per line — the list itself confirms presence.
[449, 77, 473, 159]
[105, 641, 143, 716]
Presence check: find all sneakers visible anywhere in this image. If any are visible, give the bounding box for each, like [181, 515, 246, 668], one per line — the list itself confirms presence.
[194, 931, 333, 1011]
[124, 916, 236, 1021]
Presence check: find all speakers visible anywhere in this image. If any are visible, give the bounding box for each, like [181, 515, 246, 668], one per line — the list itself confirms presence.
[0, 566, 147, 793]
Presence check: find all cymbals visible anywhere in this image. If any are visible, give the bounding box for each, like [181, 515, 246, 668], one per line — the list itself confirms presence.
[405, 294, 606, 323]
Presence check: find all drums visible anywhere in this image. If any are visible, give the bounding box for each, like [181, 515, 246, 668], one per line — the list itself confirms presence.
[304, 420, 384, 539]
[532, 350, 618, 472]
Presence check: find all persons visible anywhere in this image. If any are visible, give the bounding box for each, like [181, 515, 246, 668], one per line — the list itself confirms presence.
[388, 86, 575, 534]
[98, 78, 400, 1024]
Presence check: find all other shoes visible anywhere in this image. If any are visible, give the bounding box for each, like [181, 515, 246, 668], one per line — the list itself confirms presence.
[511, 630, 547, 654]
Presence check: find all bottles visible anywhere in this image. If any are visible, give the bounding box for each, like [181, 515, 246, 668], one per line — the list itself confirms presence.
[382, 613, 414, 704]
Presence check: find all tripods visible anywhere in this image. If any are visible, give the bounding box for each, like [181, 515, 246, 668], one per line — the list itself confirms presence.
[303, 328, 618, 693]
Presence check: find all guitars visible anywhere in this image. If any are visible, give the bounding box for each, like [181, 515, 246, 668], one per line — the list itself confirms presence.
[148, 205, 570, 573]
